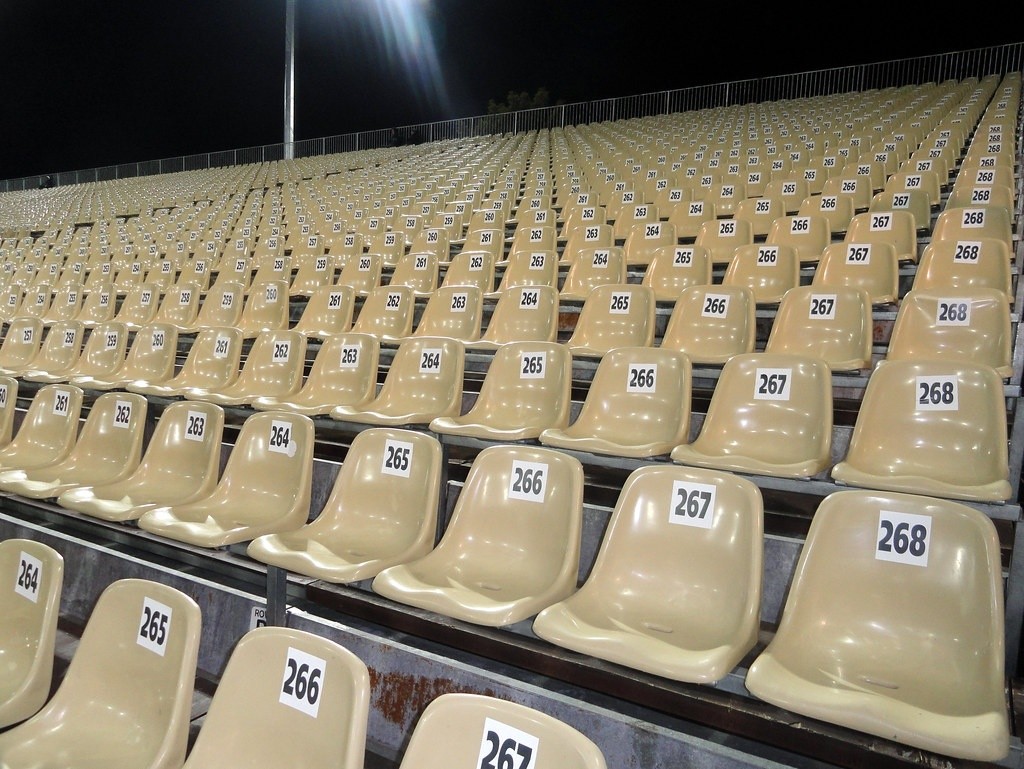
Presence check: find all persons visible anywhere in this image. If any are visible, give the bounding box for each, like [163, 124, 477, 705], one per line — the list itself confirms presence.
[38, 175, 56, 190]
[407, 129, 423, 146]
[387, 127, 403, 147]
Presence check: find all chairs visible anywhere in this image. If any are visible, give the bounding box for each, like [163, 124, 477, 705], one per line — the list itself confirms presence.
[1, 74, 1022, 768]
